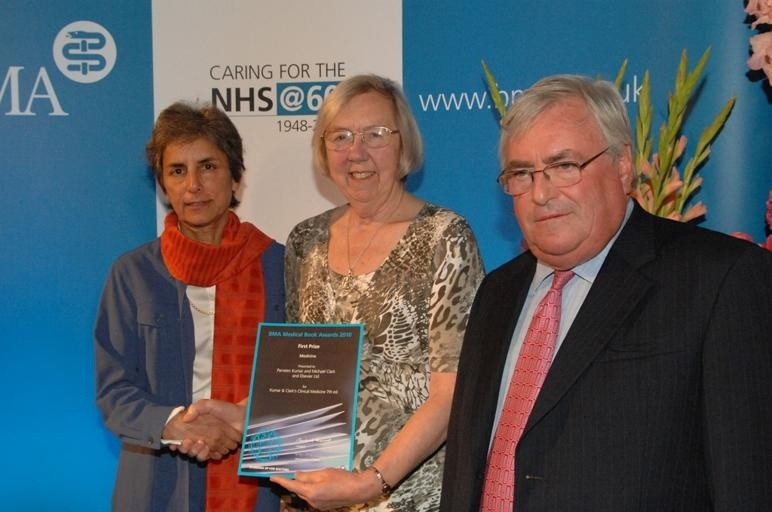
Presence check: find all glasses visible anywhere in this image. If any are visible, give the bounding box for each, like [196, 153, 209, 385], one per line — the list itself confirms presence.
[497, 148, 608, 195]
[320, 127, 399, 151]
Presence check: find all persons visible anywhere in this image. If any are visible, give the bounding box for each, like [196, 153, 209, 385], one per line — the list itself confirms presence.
[93, 95, 294, 511]
[163, 71, 490, 510]
[427, 71, 772, 512]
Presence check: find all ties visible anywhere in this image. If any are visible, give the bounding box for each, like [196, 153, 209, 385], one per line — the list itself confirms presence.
[479, 266, 575, 512]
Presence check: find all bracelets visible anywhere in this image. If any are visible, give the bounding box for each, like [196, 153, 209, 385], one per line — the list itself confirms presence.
[370, 468, 392, 497]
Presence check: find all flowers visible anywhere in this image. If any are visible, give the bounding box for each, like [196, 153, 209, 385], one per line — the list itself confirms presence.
[482, 0, 772, 255]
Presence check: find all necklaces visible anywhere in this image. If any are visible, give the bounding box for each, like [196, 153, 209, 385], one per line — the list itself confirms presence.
[342, 203, 390, 291]
